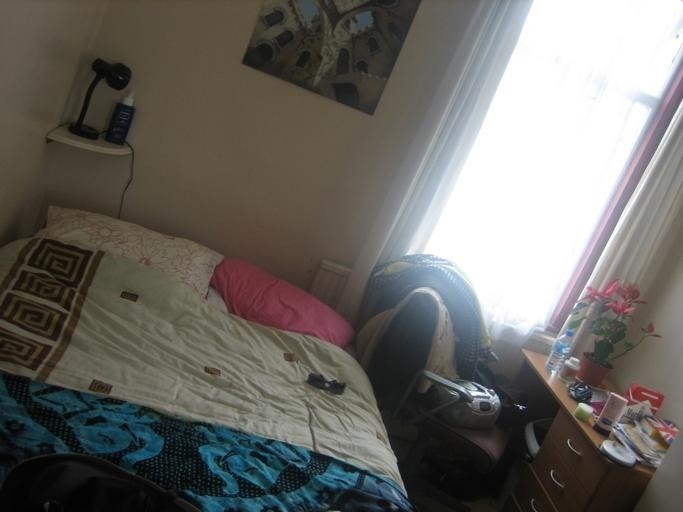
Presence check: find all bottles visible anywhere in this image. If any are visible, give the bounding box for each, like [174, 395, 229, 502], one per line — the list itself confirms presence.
[543, 330, 573, 373]
[558, 356, 580, 384]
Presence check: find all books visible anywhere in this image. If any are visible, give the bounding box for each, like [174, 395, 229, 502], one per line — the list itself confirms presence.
[600, 381, 679, 468]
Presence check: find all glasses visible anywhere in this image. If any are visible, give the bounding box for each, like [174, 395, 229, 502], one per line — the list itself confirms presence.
[307, 373, 346, 394]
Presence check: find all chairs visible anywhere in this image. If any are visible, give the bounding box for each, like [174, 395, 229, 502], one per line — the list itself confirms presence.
[386, 300, 513, 512]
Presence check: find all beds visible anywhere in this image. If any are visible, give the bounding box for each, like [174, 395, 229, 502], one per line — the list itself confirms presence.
[0, 205, 413, 510]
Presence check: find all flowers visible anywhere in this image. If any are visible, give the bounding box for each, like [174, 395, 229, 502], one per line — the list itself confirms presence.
[570, 280, 661, 362]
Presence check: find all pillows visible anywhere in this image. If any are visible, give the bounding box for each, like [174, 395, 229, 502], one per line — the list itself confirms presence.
[208, 257, 356, 353]
[34, 204, 223, 297]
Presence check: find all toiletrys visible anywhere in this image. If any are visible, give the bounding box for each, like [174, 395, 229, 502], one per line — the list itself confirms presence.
[573, 402, 594, 421]
[591, 392, 629, 438]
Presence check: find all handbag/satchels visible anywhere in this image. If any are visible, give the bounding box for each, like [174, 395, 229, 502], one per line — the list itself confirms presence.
[425, 377, 502, 429]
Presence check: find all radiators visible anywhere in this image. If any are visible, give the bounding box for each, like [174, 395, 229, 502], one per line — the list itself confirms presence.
[308, 259, 348, 310]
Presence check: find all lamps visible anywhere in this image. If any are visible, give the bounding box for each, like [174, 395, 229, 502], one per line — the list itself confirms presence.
[69, 58, 131, 139]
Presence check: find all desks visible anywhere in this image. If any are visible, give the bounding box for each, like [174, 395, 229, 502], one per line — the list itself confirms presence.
[502, 349, 657, 512]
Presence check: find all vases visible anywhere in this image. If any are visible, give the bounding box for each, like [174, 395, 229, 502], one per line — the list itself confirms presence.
[575, 351, 613, 386]
